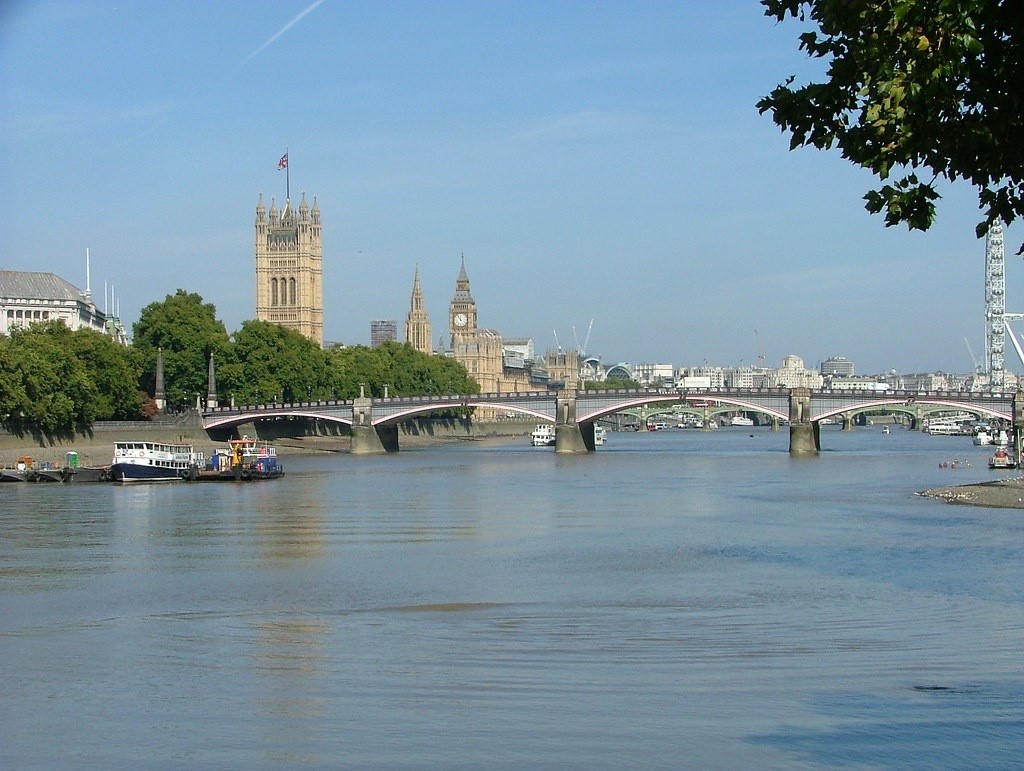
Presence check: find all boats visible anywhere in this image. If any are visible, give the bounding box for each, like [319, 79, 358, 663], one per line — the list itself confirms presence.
[110, 433, 208, 483]
[595, 427, 607, 445]
[529, 423, 556, 446]
[729, 410, 754, 427]
[1, 453, 113, 483]
[987, 443, 1018, 469]
[209, 433, 286, 479]
[972, 426, 1015, 446]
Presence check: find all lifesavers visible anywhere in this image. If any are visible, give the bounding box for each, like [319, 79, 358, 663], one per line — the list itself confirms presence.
[184, 470, 190, 477]
[116, 450, 121, 456]
[248, 470, 279, 479]
[139, 451, 144, 456]
[242, 469, 248, 477]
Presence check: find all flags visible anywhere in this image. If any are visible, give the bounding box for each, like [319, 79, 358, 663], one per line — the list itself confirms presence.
[276, 153, 288, 171]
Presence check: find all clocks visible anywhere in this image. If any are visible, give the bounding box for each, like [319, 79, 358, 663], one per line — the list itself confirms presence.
[454, 314, 468, 326]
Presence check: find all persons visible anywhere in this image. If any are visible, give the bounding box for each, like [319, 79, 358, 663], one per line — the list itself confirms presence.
[172, 409, 178, 417]
[255, 463, 265, 476]
